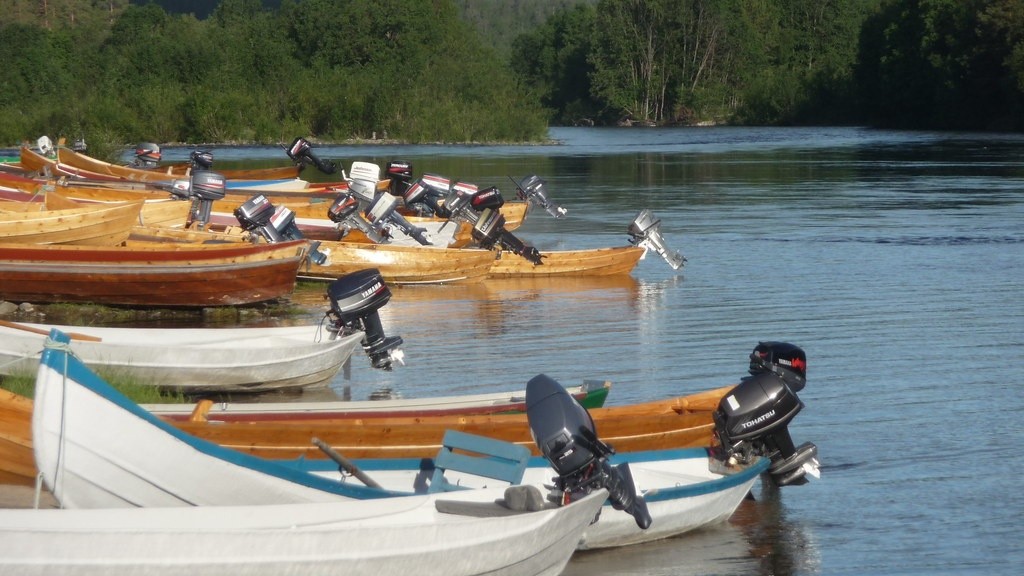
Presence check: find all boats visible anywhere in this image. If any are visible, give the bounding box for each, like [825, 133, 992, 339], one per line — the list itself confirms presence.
[1, 341, 808, 488]
[33, 328, 820, 554]
[0, 373, 651, 576]
[0, 268, 407, 394]
[0, 136, 678, 308]
